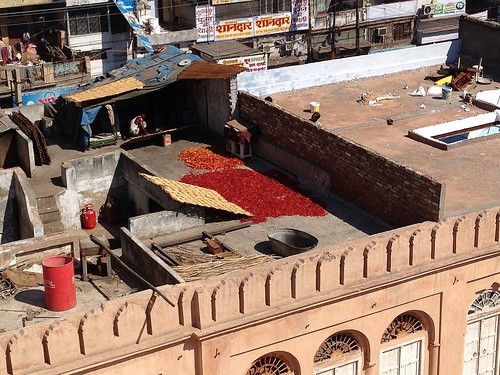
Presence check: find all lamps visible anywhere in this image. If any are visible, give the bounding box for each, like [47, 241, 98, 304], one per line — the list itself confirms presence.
[144, 5, 151, 9]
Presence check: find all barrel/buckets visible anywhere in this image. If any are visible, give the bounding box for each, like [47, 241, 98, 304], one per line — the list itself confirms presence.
[442, 87, 452, 100]
[41, 255, 77, 311]
[310, 101, 320, 113]
[267, 226, 319, 256]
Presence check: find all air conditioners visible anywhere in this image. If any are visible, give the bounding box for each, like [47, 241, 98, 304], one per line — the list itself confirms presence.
[284, 41, 293, 51]
[422, 4, 434, 15]
[374, 27, 387, 37]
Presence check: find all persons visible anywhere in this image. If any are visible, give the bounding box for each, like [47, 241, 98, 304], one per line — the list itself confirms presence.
[129, 111, 151, 137]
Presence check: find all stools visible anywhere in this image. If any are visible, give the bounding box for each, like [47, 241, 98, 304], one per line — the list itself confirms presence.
[226, 138, 252, 159]
